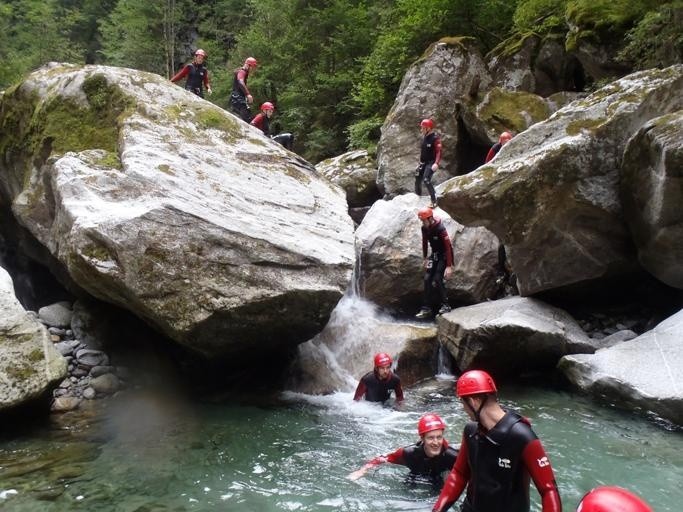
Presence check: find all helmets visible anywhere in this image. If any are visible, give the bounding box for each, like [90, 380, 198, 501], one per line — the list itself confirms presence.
[458, 370, 497, 397]
[575, 487, 651, 512]
[195, 49, 207, 57]
[375, 353, 392, 366]
[421, 120, 433, 128]
[418, 415, 444, 435]
[261, 101, 274, 110]
[244, 58, 256, 65]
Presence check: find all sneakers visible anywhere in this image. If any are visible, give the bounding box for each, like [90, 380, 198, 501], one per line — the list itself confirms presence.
[416, 310, 431, 318]
[439, 304, 451, 313]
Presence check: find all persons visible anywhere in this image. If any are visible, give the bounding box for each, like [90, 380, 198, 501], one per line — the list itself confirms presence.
[230, 57, 256, 124]
[414, 208, 454, 322]
[353, 352, 403, 404]
[414, 118, 443, 207]
[169, 48, 212, 101]
[485, 130, 514, 164]
[344, 412, 459, 483]
[247, 102, 293, 153]
[430, 369, 561, 512]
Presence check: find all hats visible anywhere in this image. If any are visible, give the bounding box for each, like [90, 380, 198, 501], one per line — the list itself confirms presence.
[418, 208, 432, 220]
